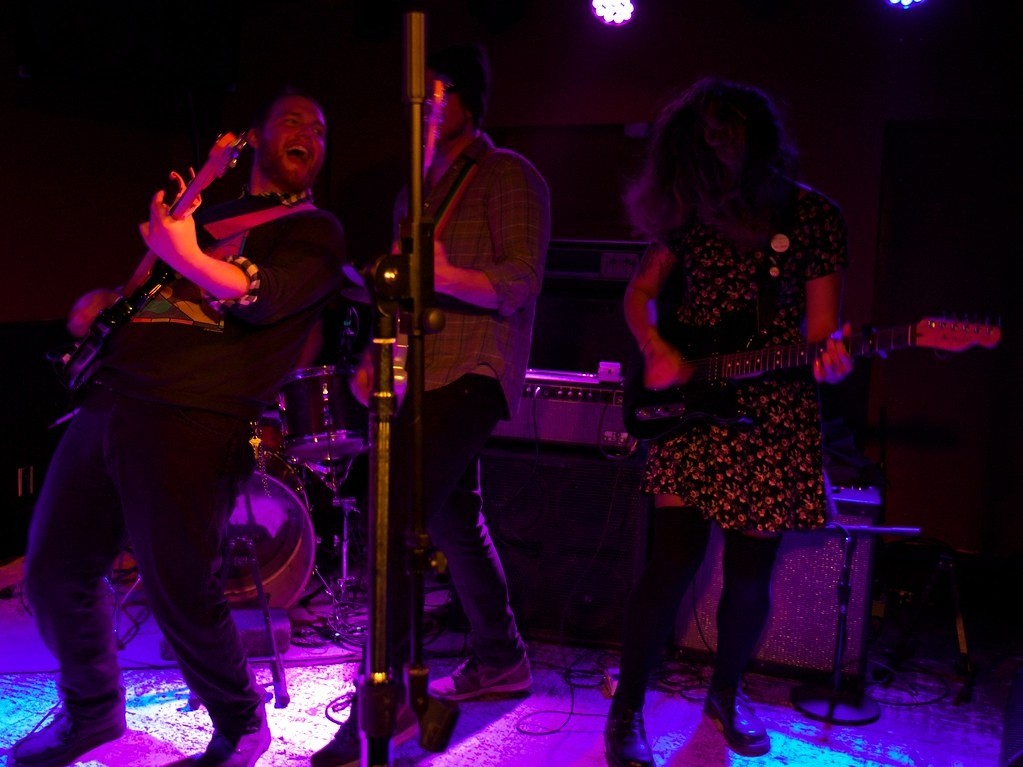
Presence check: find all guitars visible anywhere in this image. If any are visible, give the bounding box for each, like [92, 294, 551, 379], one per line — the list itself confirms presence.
[348, 77, 454, 413]
[40, 131, 247, 427]
[621, 316, 1000, 442]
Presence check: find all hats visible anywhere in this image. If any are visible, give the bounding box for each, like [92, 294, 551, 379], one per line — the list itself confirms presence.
[428, 46, 489, 128]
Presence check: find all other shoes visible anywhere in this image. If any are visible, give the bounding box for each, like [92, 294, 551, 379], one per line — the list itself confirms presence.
[197, 714, 270, 767]
[604, 707, 654, 767]
[7, 707, 126, 767]
[703, 692, 771, 754]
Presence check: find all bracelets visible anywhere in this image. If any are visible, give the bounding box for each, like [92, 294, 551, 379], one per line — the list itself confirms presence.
[639, 331, 658, 351]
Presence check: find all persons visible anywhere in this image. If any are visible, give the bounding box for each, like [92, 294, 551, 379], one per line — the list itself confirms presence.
[312, 33, 549, 767]
[13, 94, 353, 767]
[602, 77, 859, 767]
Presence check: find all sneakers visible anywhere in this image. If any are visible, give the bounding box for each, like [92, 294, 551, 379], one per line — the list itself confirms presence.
[427, 652, 533, 701]
[310, 709, 420, 767]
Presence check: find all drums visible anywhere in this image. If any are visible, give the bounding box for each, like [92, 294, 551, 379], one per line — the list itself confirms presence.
[273, 366, 373, 461]
[213, 411, 318, 612]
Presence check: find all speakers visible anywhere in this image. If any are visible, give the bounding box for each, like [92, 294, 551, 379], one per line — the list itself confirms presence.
[673, 485, 883, 685]
[480, 446, 647, 647]
